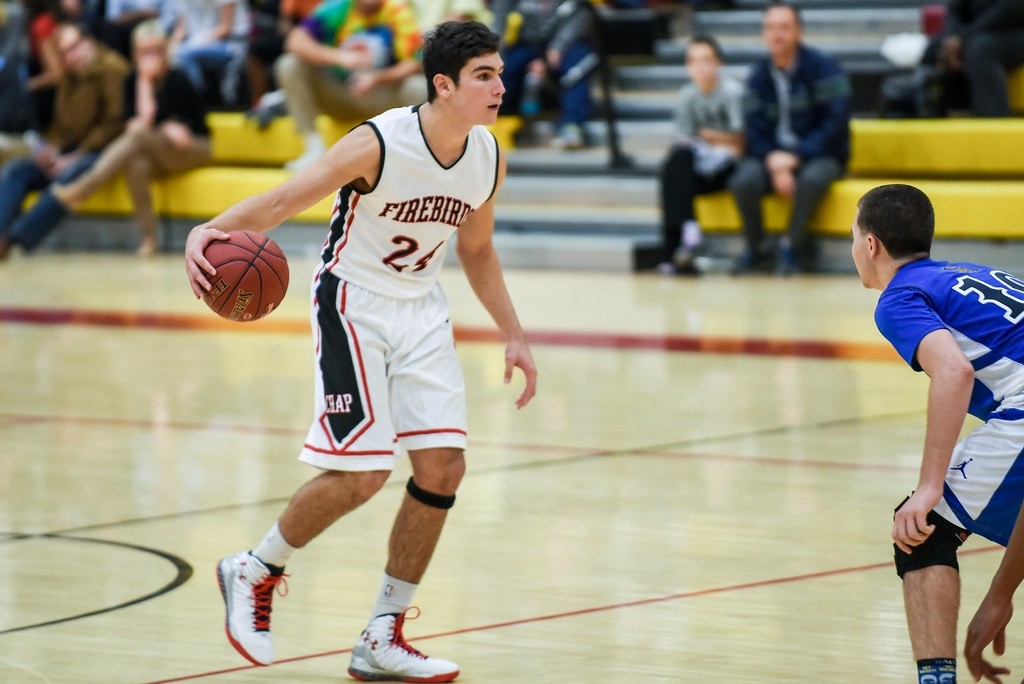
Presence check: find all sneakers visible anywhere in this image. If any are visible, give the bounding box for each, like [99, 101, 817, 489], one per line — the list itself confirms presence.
[348, 607, 460, 683]
[217, 549, 288, 667]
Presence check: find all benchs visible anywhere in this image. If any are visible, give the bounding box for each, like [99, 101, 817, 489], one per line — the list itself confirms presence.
[18, 13, 525, 254]
[695, 65, 1024, 275]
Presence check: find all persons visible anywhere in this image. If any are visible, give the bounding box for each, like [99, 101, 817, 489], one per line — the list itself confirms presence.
[656, 35, 744, 274]
[0, 0, 693, 260]
[730, 2, 853, 276]
[910, 0, 1024, 118]
[184, 22, 538, 684]
[851, 183, 1024, 684]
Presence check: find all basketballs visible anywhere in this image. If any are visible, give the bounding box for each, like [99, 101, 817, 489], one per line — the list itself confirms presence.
[202, 229, 290, 324]
